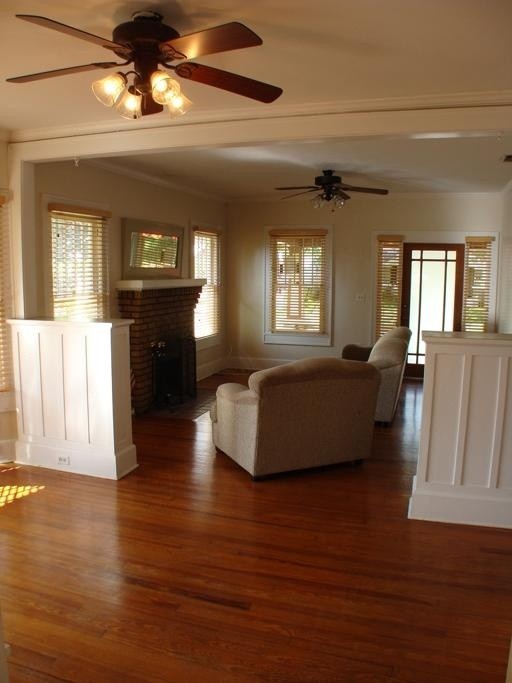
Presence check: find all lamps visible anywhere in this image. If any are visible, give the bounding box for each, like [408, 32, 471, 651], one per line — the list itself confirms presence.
[89, 67, 197, 124]
[308, 187, 349, 209]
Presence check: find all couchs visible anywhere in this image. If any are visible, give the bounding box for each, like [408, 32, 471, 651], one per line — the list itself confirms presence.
[340, 326, 414, 424]
[210, 355, 382, 481]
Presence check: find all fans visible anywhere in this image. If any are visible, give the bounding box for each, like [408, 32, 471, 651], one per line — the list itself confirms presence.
[6, 9, 285, 105]
[274, 166, 390, 209]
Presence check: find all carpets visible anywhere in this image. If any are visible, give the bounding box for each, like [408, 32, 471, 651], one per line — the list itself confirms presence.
[164, 385, 217, 420]
